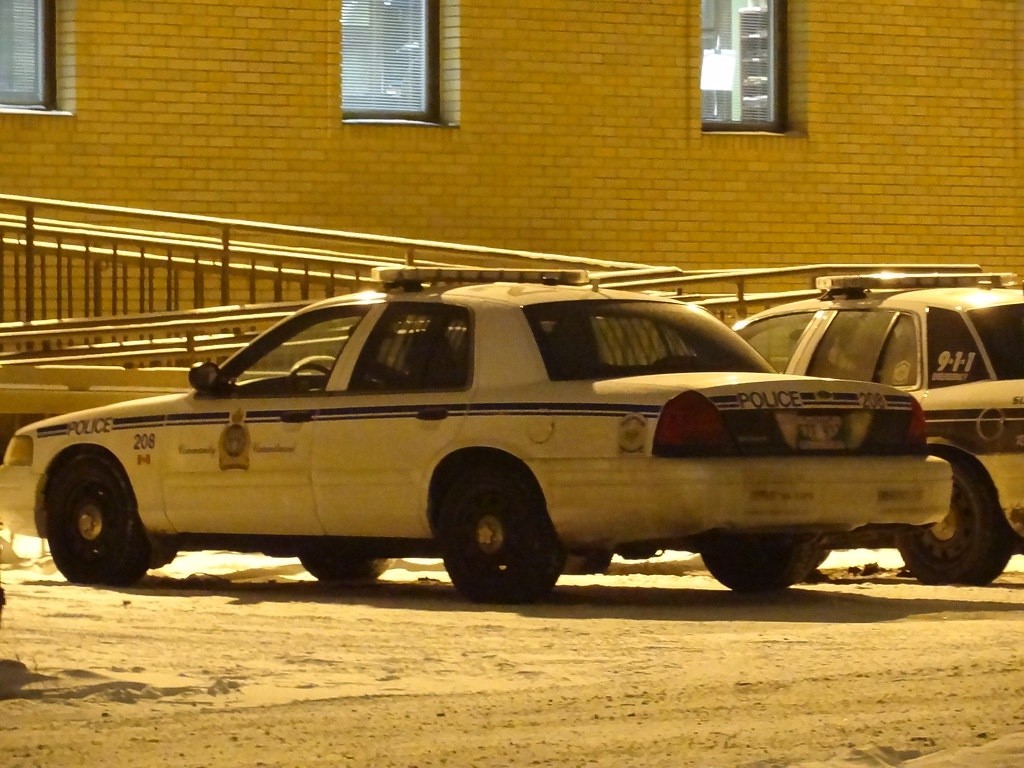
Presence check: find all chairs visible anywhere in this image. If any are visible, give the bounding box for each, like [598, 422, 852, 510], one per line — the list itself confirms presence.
[321, 321, 384, 392]
[397, 328, 444, 390]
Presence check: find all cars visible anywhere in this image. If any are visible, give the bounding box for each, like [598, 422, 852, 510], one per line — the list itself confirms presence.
[0, 265, 951, 604]
[721, 275, 1023, 585]
